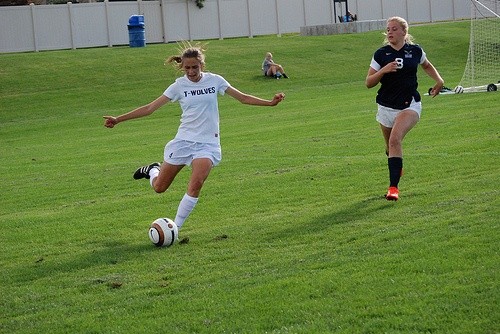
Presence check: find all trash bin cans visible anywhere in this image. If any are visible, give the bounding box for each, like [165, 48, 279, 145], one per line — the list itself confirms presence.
[127, 14, 146, 48]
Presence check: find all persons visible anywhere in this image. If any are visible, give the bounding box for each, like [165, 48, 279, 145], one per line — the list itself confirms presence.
[263, 52, 288, 79]
[103, 49, 285, 231]
[344, 11, 352, 22]
[365, 17, 444, 200]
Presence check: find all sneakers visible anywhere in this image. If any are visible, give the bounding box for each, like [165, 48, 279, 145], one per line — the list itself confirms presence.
[273, 74, 279, 79]
[133, 162, 161, 180]
[386, 186, 399, 201]
[282, 73, 290, 79]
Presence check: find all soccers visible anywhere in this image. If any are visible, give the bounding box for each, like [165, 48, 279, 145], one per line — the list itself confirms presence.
[455, 86, 463, 93]
[149, 218, 178, 247]
